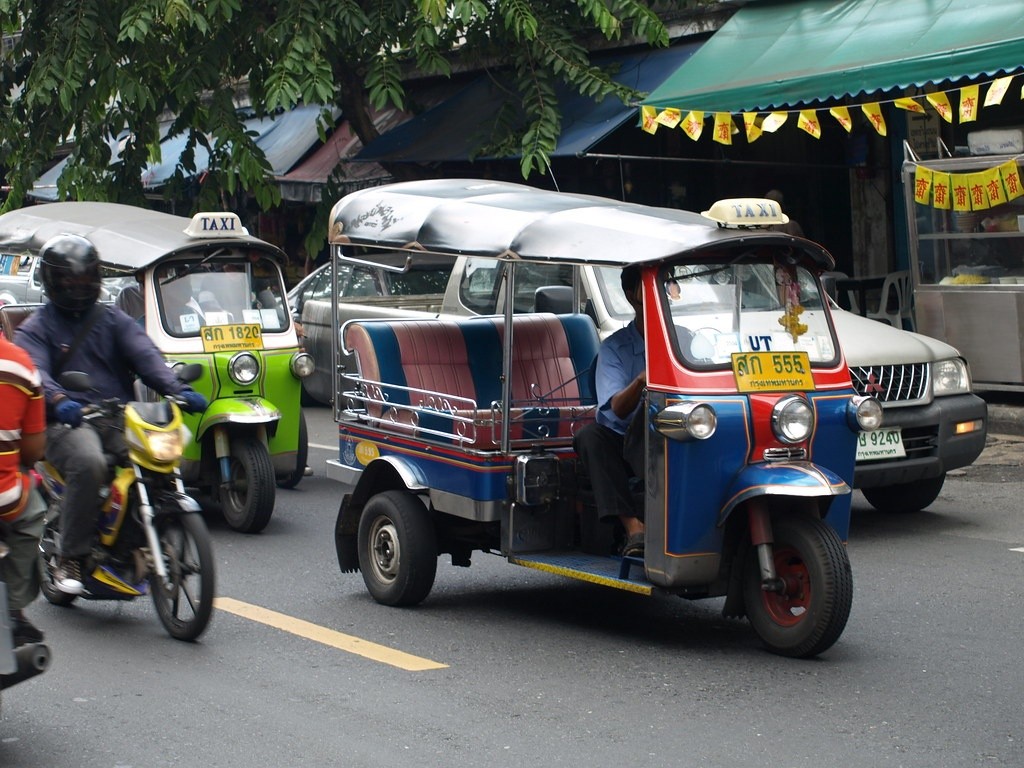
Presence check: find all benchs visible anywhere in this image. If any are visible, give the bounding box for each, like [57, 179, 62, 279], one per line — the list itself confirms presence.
[343, 314, 604, 447]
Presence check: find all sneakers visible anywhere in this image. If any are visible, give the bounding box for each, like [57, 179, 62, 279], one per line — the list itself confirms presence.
[50, 557, 83, 593]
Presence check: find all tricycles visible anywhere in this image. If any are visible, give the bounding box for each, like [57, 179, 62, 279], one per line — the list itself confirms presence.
[0, 203, 314, 535]
[323, 176, 885, 659]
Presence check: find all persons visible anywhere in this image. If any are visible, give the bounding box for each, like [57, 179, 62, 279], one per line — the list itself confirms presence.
[0, 233, 207, 640]
[114, 264, 313, 477]
[576, 263, 724, 557]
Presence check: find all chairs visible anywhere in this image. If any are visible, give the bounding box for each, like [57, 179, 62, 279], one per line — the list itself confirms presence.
[820, 269, 918, 334]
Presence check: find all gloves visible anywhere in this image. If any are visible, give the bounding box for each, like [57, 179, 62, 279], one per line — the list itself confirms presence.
[180, 391, 206, 412]
[54, 400, 83, 428]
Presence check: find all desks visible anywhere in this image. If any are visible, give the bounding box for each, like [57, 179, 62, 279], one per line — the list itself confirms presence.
[836, 276, 886, 318]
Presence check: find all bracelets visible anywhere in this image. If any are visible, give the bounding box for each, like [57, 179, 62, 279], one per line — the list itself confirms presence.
[636, 374, 646, 386]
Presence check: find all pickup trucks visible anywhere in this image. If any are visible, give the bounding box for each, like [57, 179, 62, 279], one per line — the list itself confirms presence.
[298, 224, 988, 514]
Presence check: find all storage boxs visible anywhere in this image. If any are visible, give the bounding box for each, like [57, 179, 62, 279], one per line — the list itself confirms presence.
[967, 124, 1024, 155]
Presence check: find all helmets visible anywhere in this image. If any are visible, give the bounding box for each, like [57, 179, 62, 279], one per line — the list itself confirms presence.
[40, 234, 101, 310]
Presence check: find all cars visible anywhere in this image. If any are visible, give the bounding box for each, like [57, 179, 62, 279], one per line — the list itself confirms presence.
[0, 250, 120, 307]
[271, 252, 580, 408]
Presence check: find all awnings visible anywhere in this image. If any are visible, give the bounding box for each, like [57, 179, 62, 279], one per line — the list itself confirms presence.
[26, 39, 707, 206]
[640, 0, 1024, 125]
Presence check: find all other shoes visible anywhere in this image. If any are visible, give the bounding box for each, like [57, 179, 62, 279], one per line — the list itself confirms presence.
[14, 622, 43, 645]
[623, 532, 644, 556]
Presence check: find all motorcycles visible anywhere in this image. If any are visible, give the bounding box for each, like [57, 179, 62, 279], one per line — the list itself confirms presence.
[0, 517, 53, 691]
[34, 364, 218, 642]
